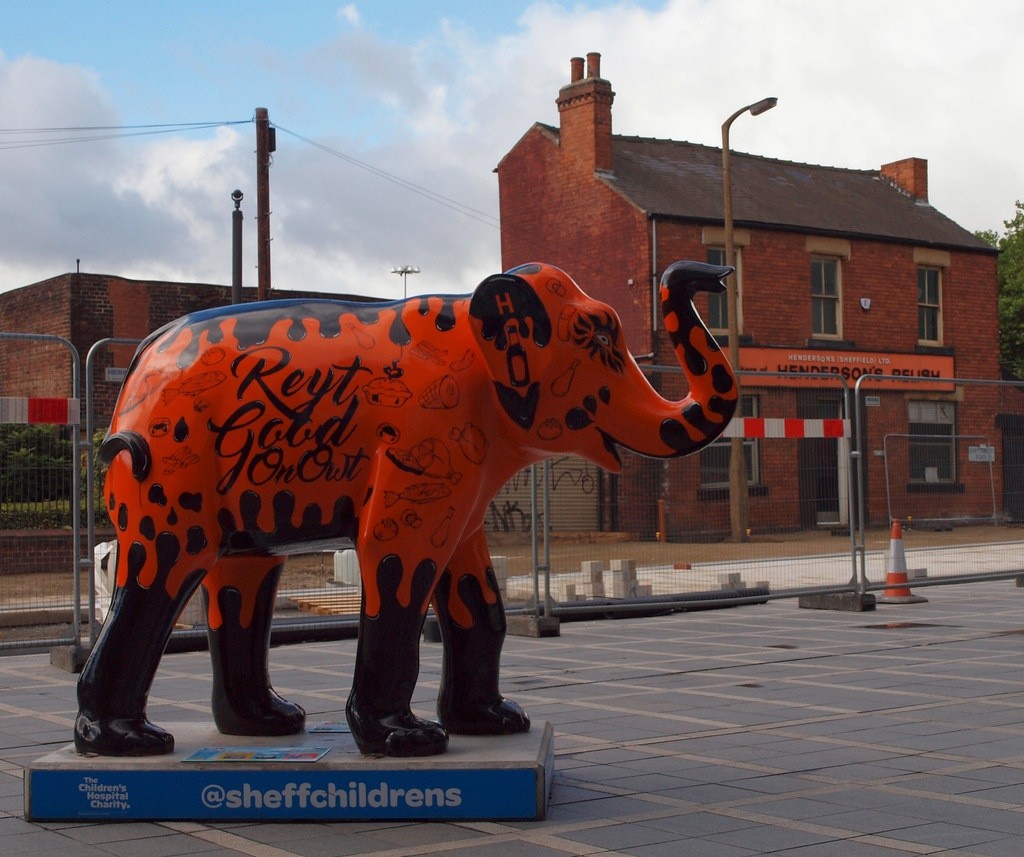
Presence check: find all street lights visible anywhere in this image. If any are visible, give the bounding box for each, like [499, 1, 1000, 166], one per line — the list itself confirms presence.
[722, 96, 780, 544]
[391, 265, 421, 299]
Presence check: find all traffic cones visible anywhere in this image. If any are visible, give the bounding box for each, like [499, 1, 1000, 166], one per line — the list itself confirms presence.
[875, 518, 930, 603]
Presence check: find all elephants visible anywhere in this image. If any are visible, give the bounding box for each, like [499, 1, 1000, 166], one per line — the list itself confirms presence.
[73, 260, 736, 755]
